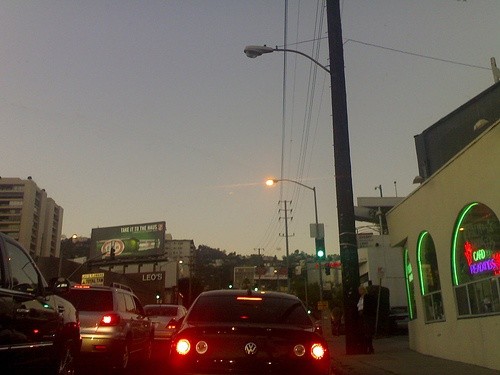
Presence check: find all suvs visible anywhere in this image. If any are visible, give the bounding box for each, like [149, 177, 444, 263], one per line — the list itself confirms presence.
[0, 233, 82, 375]
[57, 282, 154, 366]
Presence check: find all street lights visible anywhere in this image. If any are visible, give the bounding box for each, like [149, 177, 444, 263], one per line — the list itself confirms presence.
[266, 179, 325, 306]
[244, 43, 365, 356]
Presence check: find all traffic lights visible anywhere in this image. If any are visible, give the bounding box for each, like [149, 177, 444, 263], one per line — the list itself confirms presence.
[315, 238, 325, 262]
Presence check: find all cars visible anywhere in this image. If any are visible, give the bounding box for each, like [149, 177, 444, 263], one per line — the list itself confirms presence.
[169, 289, 331, 375]
[143, 303, 188, 341]
